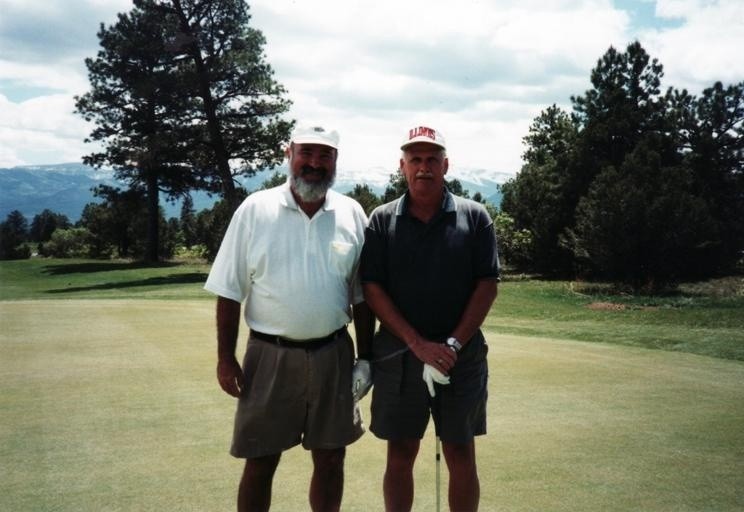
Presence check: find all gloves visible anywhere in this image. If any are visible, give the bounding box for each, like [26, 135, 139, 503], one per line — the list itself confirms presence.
[422, 363, 451, 398]
[351, 358, 374, 404]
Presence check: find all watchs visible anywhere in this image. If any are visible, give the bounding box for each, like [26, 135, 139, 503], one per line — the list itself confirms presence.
[446, 337, 462, 352]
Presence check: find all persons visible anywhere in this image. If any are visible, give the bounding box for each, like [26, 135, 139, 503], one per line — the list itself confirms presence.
[357, 126, 502, 512]
[204, 121, 377, 512]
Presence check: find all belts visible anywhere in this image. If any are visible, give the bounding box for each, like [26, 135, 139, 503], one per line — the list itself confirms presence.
[249, 325, 349, 353]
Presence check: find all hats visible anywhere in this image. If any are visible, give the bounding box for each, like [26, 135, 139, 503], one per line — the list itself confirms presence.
[399, 125, 448, 151]
[289, 117, 340, 151]
